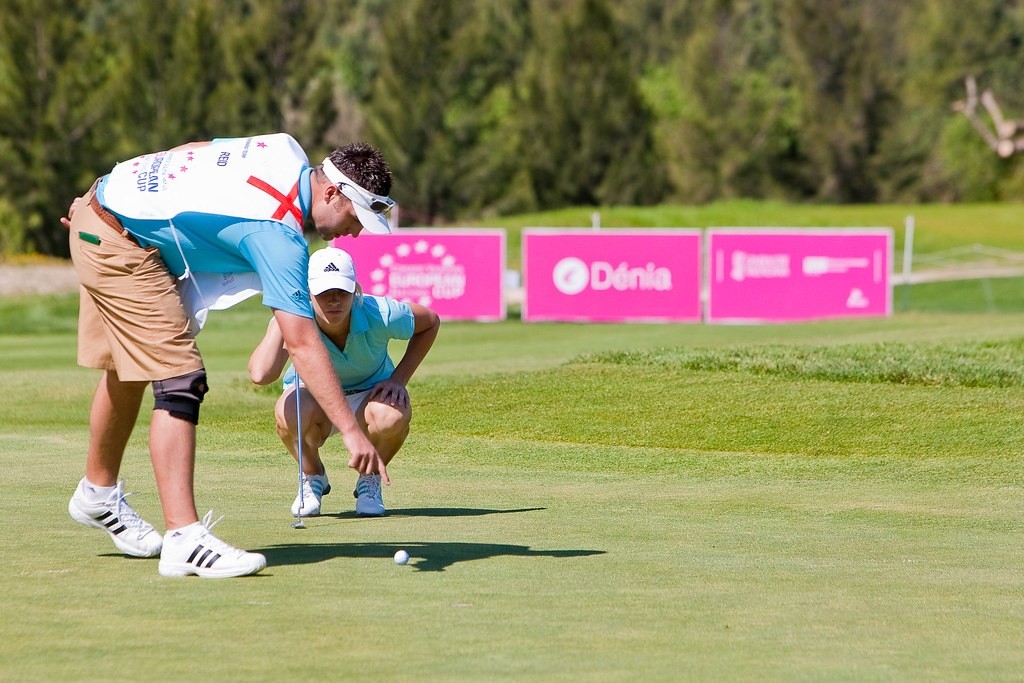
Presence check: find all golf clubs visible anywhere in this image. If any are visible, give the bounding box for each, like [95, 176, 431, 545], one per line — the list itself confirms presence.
[290, 369, 306, 528]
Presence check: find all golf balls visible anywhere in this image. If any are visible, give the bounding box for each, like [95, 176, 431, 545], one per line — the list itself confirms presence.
[394, 550, 410, 566]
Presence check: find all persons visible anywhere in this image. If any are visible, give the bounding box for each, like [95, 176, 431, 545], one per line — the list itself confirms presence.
[248, 246, 440, 519]
[61, 131, 395, 579]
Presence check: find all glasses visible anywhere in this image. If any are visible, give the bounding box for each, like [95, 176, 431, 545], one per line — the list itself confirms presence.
[337, 182, 395, 216]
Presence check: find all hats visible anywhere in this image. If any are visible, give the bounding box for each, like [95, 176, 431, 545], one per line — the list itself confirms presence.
[322, 156, 391, 235]
[307, 247, 356, 296]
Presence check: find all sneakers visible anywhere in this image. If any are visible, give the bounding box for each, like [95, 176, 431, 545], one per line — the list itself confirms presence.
[353, 471, 385, 516]
[68, 475, 164, 557]
[157, 509, 267, 578]
[292, 470, 331, 517]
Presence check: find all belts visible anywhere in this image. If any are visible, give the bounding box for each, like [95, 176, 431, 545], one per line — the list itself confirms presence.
[90, 186, 140, 247]
